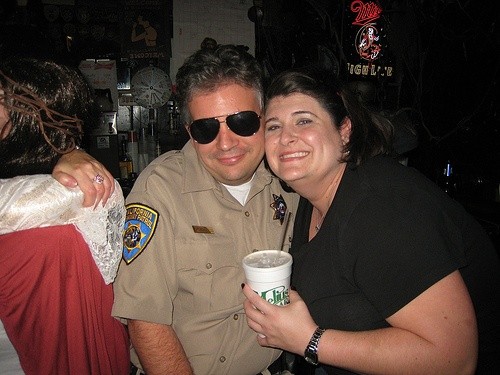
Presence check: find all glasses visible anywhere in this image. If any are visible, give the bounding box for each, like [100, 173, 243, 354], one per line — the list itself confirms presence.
[189, 111, 263, 144]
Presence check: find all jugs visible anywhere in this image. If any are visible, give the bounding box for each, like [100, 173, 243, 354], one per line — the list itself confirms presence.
[94, 88, 113, 111]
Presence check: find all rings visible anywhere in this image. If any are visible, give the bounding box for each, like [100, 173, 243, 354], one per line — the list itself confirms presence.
[92, 173, 105, 184]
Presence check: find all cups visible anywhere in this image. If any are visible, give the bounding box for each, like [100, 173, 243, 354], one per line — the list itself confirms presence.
[243, 248, 293, 336]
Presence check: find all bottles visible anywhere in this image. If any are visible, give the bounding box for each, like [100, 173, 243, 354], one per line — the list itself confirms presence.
[147, 109, 160, 158]
[128, 131, 139, 174]
[139, 126, 149, 172]
[118, 136, 133, 180]
[168, 98, 182, 135]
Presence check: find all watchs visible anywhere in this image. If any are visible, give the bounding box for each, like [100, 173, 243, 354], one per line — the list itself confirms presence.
[304, 326, 326, 365]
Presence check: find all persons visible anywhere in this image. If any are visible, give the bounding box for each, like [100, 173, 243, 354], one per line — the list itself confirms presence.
[239, 67, 480, 375]
[111, 44, 300, 374]
[1, 57, 131, 375]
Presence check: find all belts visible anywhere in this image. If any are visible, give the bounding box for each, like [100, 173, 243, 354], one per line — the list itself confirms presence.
[130, 350, 286, 375]
